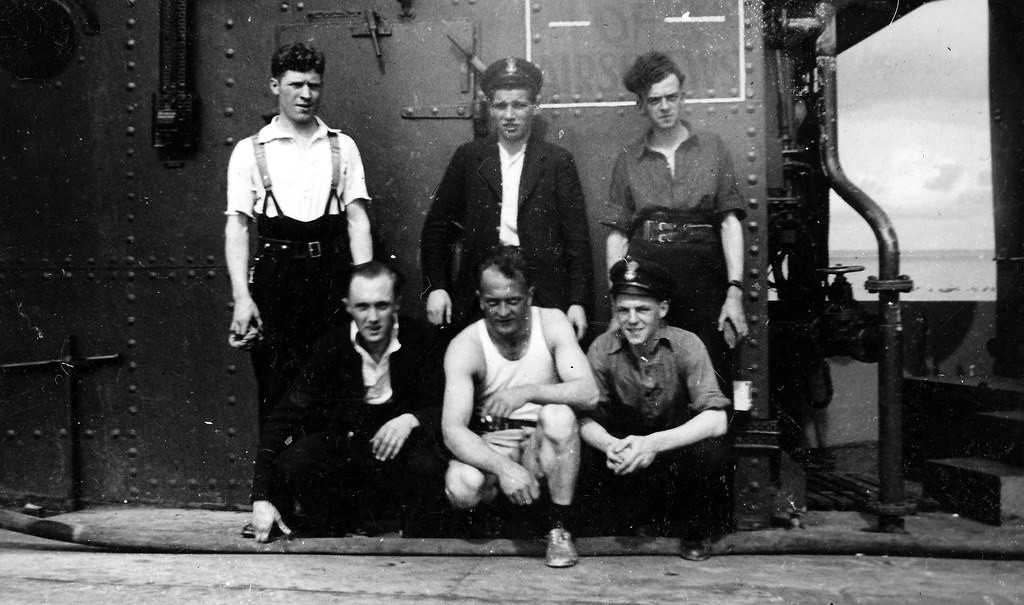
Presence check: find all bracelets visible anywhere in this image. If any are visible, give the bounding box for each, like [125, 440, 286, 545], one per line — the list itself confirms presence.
[727, 280, 745, 291]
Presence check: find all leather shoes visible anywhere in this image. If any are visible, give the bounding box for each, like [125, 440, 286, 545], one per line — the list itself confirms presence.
[680, 512, 711, 560]
[243, 488, 325, 538]
[544, 526, 578, 567]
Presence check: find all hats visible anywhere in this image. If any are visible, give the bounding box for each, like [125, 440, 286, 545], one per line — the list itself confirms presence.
[479, 57, 544, 97]
[623, 48, 672, 93]
[609, 257, 677, 304]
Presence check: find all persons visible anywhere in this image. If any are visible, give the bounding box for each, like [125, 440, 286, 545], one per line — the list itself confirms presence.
[600, 51, 748, 430]
[241, 259, 447, 543]
[421, 57, 594, 383]
[440, 254, 600, 566]
[224, 41, 375, 537]
[577, 257, 731, 561]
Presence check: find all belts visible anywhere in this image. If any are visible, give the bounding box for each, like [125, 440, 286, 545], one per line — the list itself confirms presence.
[633, 209, 718, 243]
[254, 232, 348, 261]
